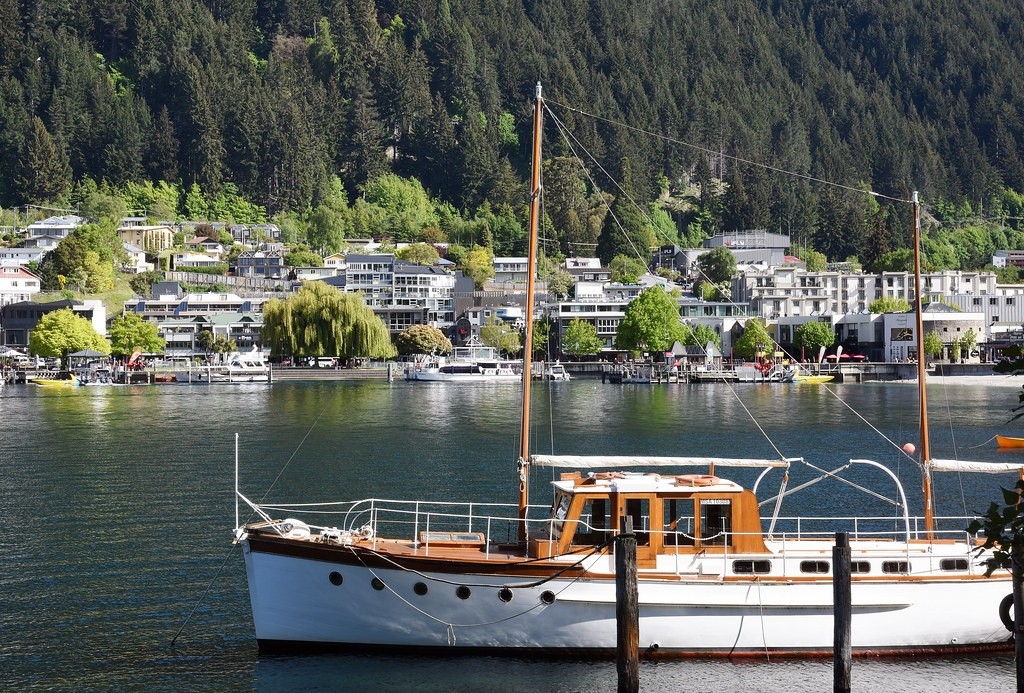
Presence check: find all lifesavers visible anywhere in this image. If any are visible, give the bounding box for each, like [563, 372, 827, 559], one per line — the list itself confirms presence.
[674, 474, 720, 486]
[591, 472, 623, 480]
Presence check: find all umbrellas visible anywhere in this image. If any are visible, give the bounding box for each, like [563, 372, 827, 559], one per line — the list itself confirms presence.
[826, 354, 865, 361]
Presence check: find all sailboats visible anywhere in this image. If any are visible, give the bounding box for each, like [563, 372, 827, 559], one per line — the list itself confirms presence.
[233, 82, 1024, 665]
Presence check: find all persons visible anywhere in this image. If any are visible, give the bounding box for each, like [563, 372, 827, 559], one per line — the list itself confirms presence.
[790, 357, 816, 369]
[281, 357, 363, 368]
[895, 356, 916, 364]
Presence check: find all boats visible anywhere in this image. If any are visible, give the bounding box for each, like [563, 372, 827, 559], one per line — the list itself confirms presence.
[403, 329, 571, 383]
[30, 373, 81, 389]
[994, 434, 1024, 450]
[173, 343, 272, 383]
[733, 340, 835, 385]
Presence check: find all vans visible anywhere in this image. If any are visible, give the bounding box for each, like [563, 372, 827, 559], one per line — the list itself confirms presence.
[307, 356, 341, 370]
